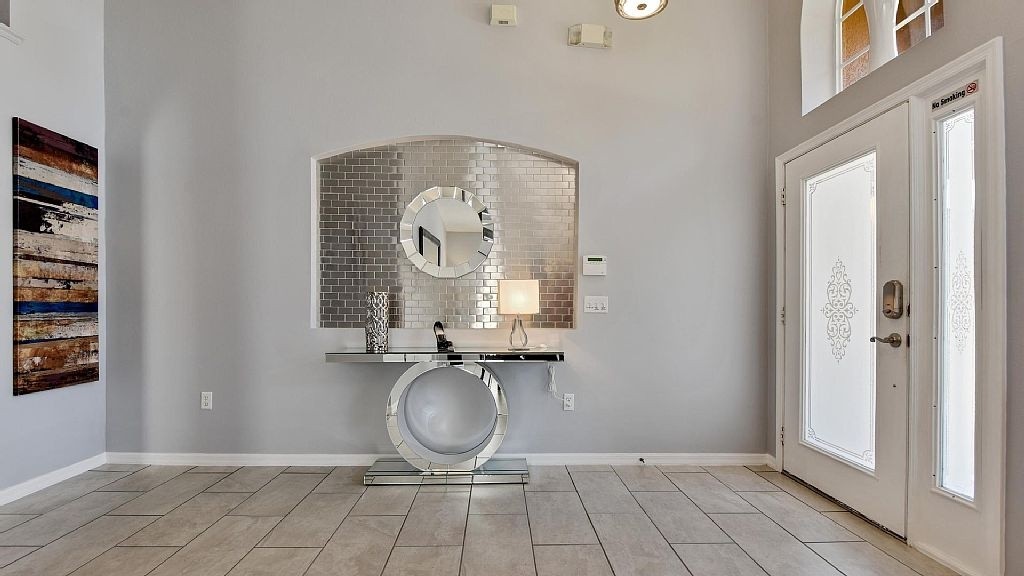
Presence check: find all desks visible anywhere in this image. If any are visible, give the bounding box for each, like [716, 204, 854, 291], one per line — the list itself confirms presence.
[326, 347, 565, 485]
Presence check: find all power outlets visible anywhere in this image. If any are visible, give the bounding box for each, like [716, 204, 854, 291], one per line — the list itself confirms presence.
[564, 393, 574, 410]
[200, 392, 212, 409]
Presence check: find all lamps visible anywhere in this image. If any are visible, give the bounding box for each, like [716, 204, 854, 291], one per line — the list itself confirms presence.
[567, 24, 614, 48]
[614, 0, 669, 20]
[497, 279, 541, 350]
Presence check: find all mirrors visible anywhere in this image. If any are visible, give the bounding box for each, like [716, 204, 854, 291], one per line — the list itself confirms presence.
[400, 187, 495, 279]
